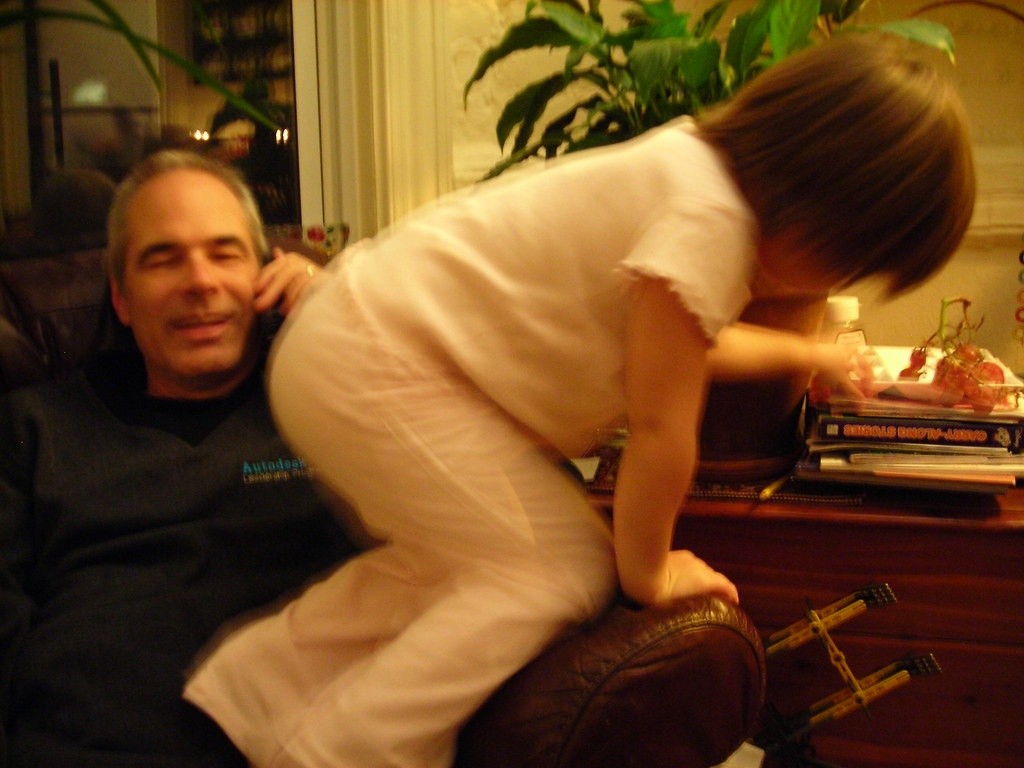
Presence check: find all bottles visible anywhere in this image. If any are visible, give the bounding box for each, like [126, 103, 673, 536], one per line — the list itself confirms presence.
[808, 296, 874, 409]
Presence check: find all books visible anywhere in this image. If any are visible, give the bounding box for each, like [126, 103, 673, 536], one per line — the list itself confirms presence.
[794, 345, 1024, 495]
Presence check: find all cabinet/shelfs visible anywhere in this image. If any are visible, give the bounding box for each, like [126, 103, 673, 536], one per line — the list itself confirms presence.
[591, 459, 1024, 768]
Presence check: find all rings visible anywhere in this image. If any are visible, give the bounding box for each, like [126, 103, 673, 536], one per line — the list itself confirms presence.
[307, 263, 316, 278]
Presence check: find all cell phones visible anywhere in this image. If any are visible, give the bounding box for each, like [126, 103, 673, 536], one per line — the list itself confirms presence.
[263, 254, 284, 342]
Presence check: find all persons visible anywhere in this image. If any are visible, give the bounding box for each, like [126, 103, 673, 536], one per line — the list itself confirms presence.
[175, 30, 979, 767]
[16, 58, 301, 260]
[0, 148, 355, 768]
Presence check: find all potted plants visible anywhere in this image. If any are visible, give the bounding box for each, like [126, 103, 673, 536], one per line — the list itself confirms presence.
[464, 0, 959, 481]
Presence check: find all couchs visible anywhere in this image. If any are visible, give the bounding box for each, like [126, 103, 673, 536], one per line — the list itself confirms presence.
[0, 240, 766, 768]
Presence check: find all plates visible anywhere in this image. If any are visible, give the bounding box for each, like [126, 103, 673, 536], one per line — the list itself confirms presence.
[857, 345, 1024, 403]
